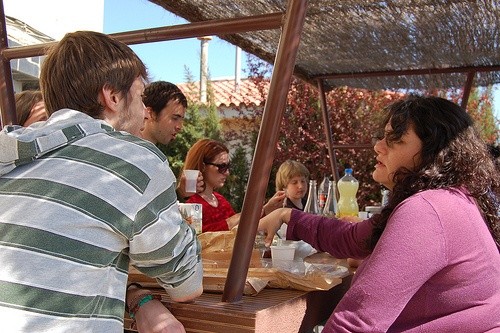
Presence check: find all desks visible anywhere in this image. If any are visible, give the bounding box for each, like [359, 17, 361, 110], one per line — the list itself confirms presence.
[124, 252, 357, 333]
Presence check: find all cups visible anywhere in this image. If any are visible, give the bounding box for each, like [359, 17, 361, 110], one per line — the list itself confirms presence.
[183, 169, 200, 193]
[270, 244, 295, 272]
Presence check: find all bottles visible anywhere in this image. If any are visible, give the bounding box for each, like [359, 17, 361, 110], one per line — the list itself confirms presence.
[336, 168, 360, 219]
[324, 180, 338, 217]
[315, 172, 331, 211]
[303, 180, 319, 213]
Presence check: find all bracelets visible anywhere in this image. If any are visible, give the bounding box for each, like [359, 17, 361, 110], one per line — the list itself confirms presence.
[125, 282, 162, 320]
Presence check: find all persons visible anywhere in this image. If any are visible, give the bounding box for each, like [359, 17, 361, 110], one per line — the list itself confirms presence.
[0, 31, 204, 333]
[137, 80, 204, 203]
[257, 94, 500, 333]
[180, 139, 289, 233]
[13, 91, 48, 127]
[275, 159, 309, 211]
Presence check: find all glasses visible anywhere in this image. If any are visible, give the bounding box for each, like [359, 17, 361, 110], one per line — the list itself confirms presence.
[202, 160, 233, 175]
